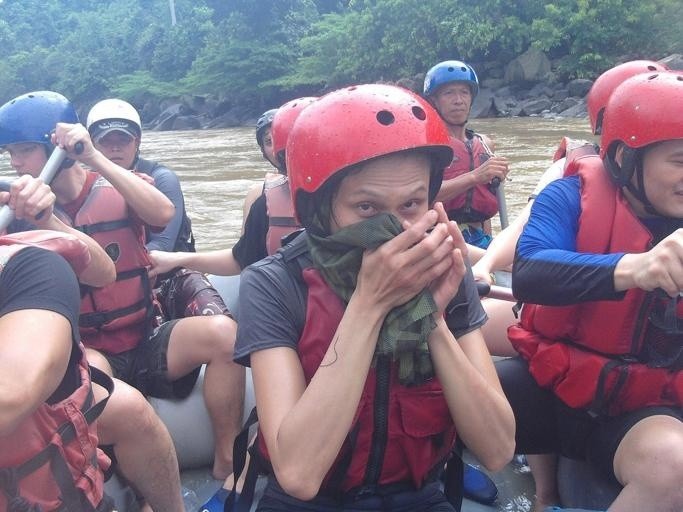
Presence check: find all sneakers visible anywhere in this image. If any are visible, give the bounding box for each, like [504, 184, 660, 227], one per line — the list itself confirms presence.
[440, 457, 498, 504]
[198, 484, 242, 512]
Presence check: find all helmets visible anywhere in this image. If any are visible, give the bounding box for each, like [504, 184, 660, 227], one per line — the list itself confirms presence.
[0, 90, 81, 162]
[254, 108, 279, 153]
[86, 98, 144, 132]
[271, 97, 320, 162]
[285, 83, 454, 232]
[585, 58, 669, 136]
[599, 69, 683, 185]
[422, 58, 480, 98]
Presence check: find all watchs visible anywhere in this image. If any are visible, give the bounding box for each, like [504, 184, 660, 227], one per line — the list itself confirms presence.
[0, 90, 250, 479]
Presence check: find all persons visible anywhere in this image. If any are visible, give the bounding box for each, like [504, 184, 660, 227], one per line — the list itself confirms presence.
[241, 109, 287, 226]
[84, 100, 234, 323]
[422, 59, 508, 251]
[486, 71, 683, 511]
[0, 174, 184, 511]
[1, 240, 119, 511]
[222, 84, 517, 510]
[137, 96, 336, 277]
[475, 58, 670, 354]
